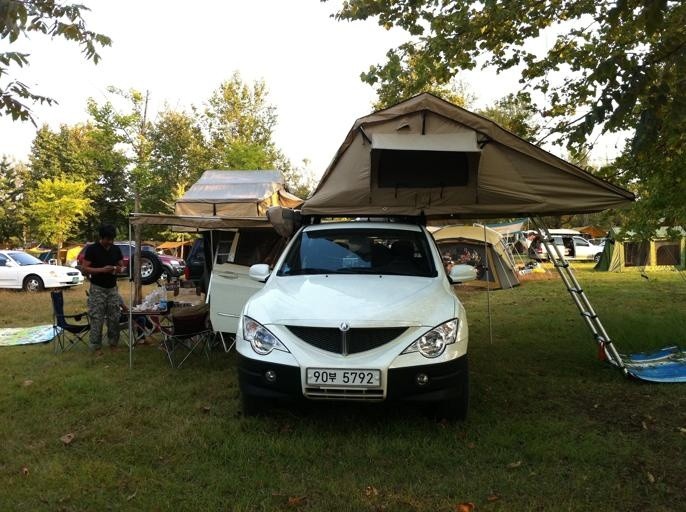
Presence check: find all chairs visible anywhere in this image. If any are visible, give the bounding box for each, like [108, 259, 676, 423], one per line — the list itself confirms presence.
[49, 290, 91, 355]
[157, 299, 216, 370]
[317, 239, 418, 271]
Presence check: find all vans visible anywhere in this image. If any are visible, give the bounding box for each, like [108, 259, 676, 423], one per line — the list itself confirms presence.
[526, 234, 604, 263]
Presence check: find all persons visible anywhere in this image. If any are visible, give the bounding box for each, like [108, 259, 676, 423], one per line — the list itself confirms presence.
[115, 286, 162, 346]
[82, 226, 127, 360]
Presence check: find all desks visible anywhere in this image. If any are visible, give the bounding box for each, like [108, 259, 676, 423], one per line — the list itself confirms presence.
[120, 308, 174, 352]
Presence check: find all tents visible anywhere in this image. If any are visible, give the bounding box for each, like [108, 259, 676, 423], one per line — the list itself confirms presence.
[432, 221, 521, 292]
[594, 225, 685, 272]
[300, 91, 637, 221]
[171, 168, 305, 233]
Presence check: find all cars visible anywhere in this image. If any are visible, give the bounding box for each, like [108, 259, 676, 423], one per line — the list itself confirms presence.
[0, 250, 84, 294]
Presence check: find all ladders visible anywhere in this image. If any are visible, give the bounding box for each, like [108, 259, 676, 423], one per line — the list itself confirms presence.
[529, 214, 629, 376]
[205, 232, 241, 304]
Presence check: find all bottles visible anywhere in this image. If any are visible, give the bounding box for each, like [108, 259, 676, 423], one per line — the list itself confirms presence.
[160, 279, 168, 312]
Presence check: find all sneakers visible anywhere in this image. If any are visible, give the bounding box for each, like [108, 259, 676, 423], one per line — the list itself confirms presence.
[94, 346, 104, 358]
[110, 345, 121, 352]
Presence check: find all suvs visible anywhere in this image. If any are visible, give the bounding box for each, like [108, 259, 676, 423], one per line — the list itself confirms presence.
[198, 217, 480, 422]
[75, 241, 188, 284]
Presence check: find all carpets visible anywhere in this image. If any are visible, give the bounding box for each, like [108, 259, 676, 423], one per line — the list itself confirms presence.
[0, 324, 62, 347]
